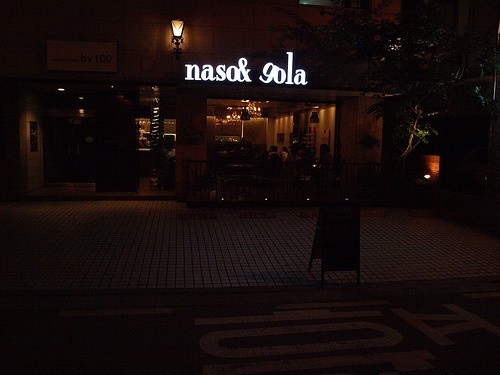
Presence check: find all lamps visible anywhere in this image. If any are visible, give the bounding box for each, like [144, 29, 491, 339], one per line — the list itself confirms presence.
[168, 15, 186, 58]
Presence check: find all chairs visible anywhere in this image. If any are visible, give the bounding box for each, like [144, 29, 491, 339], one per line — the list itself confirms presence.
[206, 142, 332, 200]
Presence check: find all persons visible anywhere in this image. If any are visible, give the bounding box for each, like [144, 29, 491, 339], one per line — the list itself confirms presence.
[241, 144, 294, 166]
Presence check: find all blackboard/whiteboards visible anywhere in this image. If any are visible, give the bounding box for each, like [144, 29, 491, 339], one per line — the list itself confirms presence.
[310, 205, 363, 272]
